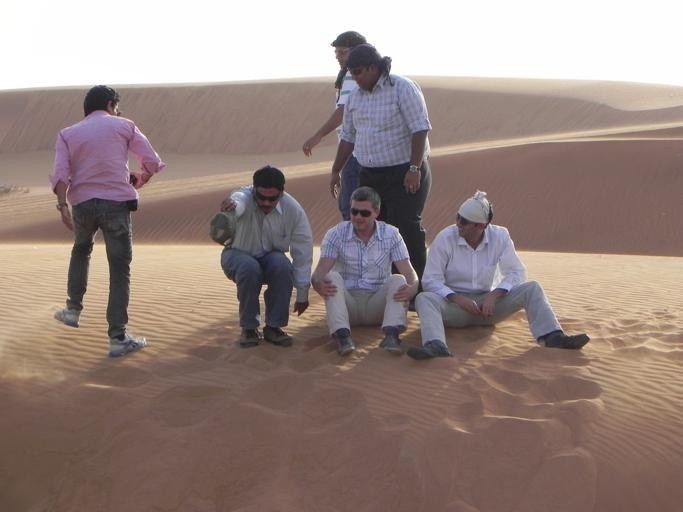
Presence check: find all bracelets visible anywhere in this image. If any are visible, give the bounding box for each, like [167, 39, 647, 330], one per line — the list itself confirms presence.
[56, 203, 67, 211]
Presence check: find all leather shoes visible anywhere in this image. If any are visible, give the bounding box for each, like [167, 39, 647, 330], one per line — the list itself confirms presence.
[545, 333, 590, 350]
[407, 344, 454, 360]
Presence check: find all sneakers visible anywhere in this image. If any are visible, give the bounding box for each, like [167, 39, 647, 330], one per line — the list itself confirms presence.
[379, 336, 402, 354]
[54, 308, 81, 328]
[336, 332, 356, 356]
[239, 325, 261, 347]
[109, 333, 147, 357]
[263, 326, 293, 348]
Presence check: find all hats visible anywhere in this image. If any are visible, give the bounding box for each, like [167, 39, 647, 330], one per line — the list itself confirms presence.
[331, 32, 366, 47]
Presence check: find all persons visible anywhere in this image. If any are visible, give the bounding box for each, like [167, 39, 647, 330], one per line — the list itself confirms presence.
[302, 31, 367, 221]
[47, 86, 166, 357]
[220, 166, 314, 348]
[311, 187, 420, 357]
[406, 189, 591, 361]
[330, 43, 432, 311]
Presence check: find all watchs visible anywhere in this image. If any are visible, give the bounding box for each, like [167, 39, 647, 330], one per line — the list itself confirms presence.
[409, 165, 420, 173]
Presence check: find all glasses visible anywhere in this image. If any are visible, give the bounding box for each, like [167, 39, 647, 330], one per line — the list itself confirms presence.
[457, 213, 468, 224]
[350, 208, 372, 217]
[349, 65, 369, 76]
[256, 192, 279, 201]
